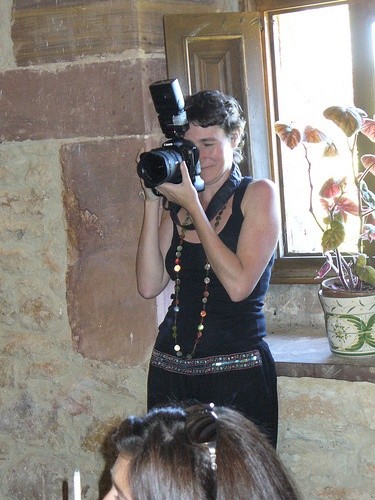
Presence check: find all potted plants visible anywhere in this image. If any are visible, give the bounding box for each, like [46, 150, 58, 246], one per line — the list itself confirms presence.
[274, 103, 375, 361]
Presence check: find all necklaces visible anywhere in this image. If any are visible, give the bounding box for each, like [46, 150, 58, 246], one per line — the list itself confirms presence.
[171, 202, 226, 360]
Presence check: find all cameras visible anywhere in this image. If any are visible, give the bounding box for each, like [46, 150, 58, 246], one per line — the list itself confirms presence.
[136, 77, 205, 197]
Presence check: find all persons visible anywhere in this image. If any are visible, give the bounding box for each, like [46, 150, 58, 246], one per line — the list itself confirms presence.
[99, 403, 304, 500]
[135, 91, 281, 455]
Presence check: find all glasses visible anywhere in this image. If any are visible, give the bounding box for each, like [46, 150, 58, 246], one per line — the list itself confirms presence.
[185, 400, 220, 482]
